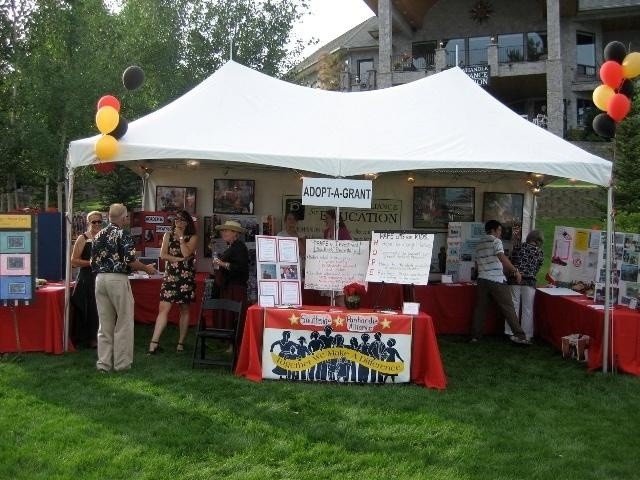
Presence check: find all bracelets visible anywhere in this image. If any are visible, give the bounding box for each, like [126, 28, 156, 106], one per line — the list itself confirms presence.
[179, 237, 183, 240]
[511, 268, 519, 273]
[225, 262, 230, 270]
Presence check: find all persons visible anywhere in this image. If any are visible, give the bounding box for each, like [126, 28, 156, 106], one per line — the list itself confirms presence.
[212, 220, 250, 353]
[323, 209, 352, 240]
[501, 230, 545, 347]
[438, 246, 447, 273]
[270, 324, 405, 384]
[71, 211, 104, 351]
[88, 203, 159, 376]
[466, 219, 528, 345]
[145, 209, 200, 356]
[276, 211, 308, 259]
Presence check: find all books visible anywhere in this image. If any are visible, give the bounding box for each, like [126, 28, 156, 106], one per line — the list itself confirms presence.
[588, 304, 616, 311]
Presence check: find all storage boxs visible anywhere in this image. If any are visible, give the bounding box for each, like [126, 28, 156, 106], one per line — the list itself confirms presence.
[562, 333, 589, 364]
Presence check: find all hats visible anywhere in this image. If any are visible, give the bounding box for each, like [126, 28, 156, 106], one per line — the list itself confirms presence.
[214, 221, 249, 234]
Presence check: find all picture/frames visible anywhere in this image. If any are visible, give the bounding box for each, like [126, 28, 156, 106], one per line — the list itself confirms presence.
[483, 190, 524, 247]
[155, 185, 197, 214]
[212, 178, 255, 215]
[428, 231, 449, 281]
[137, 256, 159, 272]
[203, 216, 215, 257]
[412, 185, 475, 230]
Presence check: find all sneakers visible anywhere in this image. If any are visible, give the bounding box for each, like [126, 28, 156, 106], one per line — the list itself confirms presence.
[471, 338, 480, 344]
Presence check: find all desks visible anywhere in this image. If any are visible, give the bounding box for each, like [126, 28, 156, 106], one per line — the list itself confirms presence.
[532, 284, 640, 377]
[254, 279, 405, 308]
[233, 303, 448, 390]
[0, 278, 76, 354]
[409, 278, 535, 335]
[122, 270, 217, 327]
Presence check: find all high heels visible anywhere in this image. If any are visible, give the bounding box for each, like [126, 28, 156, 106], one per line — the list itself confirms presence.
[176, 342, 185, 352]
[146, 341, 160, 356]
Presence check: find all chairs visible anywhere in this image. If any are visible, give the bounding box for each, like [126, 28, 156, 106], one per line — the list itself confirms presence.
[521, 114, 547, 130]
[191, 295, 242, 375]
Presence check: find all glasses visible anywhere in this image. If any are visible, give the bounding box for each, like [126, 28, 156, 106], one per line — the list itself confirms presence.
[90, 220, 102, 224]
[174, 216, 186, 221]
[126, 216, 128, 217]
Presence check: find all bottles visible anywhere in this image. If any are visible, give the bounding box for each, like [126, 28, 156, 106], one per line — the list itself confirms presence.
[213, 252, 219, 270]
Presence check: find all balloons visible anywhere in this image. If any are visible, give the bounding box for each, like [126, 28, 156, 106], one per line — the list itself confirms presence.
[95, 135, 119, 162]
[621, 52, 640, 79]
[606, 92, 630, 121]
[95, 106, 120, 134]
[122, 66, 145, 92]
[592, 113, 616, 138]
[95, 94, 120, 114]
[106, 116, 129, 140]
[603, 41, 626, 65]
[599, 61, 624, 90]
[95, 163, 115, 174]
[592, 85, 616, 111]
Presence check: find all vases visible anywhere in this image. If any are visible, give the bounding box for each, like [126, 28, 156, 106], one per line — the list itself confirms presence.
[344, 294, 361, 309]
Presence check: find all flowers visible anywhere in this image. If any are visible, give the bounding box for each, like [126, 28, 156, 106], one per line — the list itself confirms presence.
[343, 283, 367, 297]
